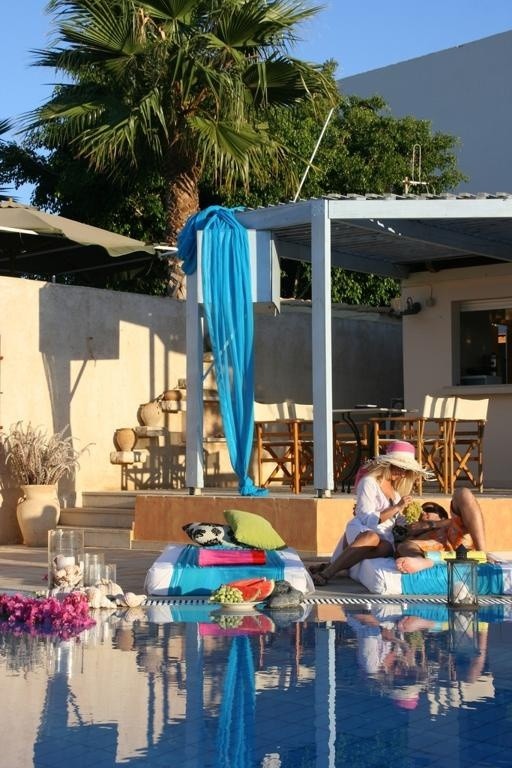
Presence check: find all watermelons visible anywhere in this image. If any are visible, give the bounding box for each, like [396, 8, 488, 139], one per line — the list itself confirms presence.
[229, 576, 274, 602]
[239, 615, 275, 633]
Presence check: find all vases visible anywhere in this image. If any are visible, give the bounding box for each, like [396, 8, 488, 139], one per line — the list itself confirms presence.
[115, 402, 161, 451]
[14, 483, 61, 547]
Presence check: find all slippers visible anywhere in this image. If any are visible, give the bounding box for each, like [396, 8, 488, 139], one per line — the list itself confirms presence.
[308, 563, 326, 574]
[314, 573, 329, 586]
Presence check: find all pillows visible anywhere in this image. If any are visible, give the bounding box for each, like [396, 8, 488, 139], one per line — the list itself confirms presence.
[180, 509, 285, 565]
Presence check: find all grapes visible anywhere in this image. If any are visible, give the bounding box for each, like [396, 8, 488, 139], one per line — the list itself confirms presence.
[212, 615, 243, 628]
[402, 501, 424, 526]
[211, 585, 243, 604]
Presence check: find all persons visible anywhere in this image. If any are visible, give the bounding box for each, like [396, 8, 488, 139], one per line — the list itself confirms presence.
[393, 615, 490, 686]
[345, 614, 436, 710]
[308, 440, 436, 586]
[352, 487, 489, 574]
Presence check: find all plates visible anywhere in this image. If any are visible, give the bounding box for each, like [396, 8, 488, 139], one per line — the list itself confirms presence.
[217, 600, 264, 610]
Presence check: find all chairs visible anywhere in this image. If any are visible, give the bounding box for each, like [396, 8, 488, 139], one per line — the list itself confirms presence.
[245, 393, 490, 495]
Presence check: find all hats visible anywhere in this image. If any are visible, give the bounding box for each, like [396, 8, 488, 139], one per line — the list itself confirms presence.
[377, 440, 431, 475]
[383, 687, 421, 710]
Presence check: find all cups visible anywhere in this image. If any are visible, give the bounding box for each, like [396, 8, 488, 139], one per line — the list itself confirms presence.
[48, 528, 116, 589]
